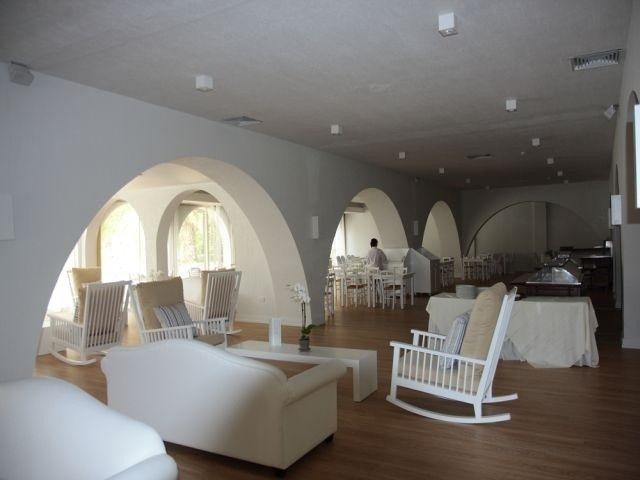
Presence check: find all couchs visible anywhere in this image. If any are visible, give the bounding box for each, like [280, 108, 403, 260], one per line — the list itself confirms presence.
[0, 377, 178, 480]
[100, 338, 349, 478]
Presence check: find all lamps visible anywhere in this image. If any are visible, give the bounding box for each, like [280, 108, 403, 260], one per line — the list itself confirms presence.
[605, 104, 619, 120]
[399, 151, 406, 160]
[330, 124, 342, 136]
[438, 13, 459, 39]
[196, 75, 215, 91]
[506, 97, 518, 112]
[532, 138, 540, 147]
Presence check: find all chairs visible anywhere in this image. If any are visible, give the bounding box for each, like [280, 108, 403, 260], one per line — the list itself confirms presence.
[131, 280, 228, 352]
[439, 255, 509, 286]
[182, 268, 244, 337]
[323, 255, 416, 315]
[48, 279, 132, 367]
[387, 284, 518, 423]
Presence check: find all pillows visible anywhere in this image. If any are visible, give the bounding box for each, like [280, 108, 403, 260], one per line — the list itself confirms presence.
[71, 265, 103, 325]
[157, 304, 198, 340]
[439, 311, 470, 372]
[460, 282, 509, 379]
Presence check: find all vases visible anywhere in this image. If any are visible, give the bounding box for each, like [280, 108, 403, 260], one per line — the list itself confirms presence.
[297, 337, 312, 353]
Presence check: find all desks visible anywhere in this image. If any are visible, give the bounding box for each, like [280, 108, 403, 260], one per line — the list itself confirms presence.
[526, 255, 586, 297]
[425, 294, 600, 368]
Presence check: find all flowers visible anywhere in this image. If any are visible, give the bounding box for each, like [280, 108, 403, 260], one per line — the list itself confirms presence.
[288, 284, 318, 340]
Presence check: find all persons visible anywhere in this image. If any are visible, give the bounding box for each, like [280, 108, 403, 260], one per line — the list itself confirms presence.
[366, 238, 387, 305]
